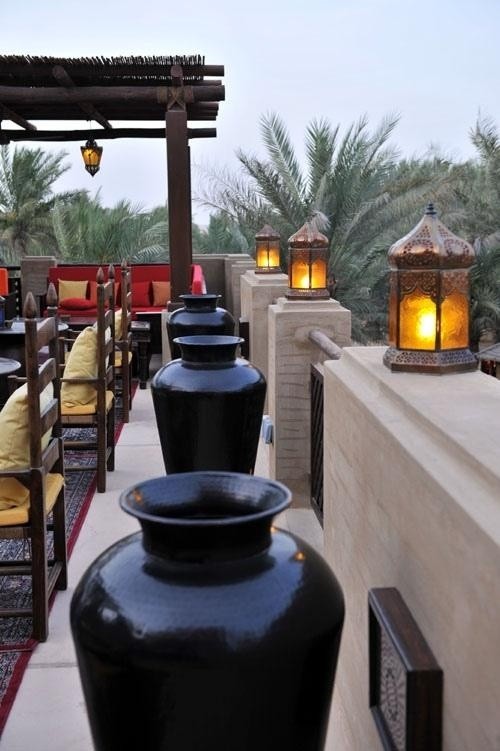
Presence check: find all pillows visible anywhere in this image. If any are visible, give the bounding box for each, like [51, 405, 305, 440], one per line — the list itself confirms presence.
[132, 280, 171, 308]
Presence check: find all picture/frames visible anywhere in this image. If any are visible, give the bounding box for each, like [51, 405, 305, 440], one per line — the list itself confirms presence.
[363, 586, 443, 751]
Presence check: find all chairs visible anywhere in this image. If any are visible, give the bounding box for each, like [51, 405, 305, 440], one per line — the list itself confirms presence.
[0, 284, 71, 646]
[53, 258, 134, 494]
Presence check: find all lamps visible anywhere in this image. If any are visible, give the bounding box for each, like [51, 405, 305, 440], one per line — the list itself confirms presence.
[253, 215, 334, 302]
[380, 202, 481, 378]
[79, 117, 103, 178]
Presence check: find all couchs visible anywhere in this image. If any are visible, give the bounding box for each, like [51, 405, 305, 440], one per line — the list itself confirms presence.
[43, 266, 205, 352]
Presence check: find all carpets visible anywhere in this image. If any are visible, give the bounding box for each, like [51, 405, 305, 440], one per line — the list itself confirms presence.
[0, 380, 141, 739]
